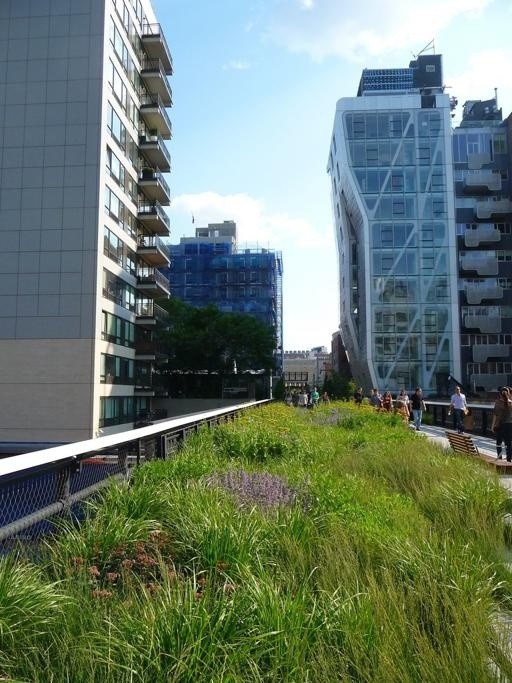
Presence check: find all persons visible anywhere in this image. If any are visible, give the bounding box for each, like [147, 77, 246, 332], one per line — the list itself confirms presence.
[410, 386, 427, 430]
[491, 386, 512, 462]
[396, 388, 410, 422]
[291, 385, 330, 408]
[448, 385, 470, 435]
[354, 386, 394, 411]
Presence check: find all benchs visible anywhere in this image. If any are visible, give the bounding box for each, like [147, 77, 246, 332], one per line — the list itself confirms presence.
[446, 430, 512, 478]
